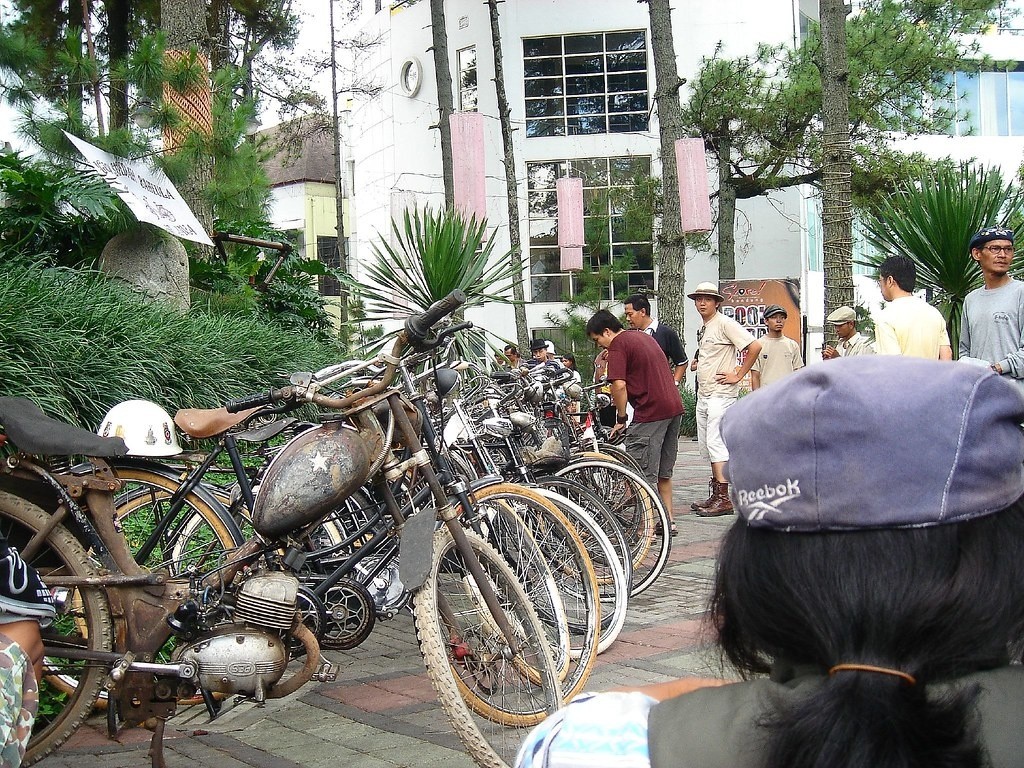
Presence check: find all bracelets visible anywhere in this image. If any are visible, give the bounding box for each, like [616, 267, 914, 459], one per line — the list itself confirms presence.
[617, 413, 628, 424]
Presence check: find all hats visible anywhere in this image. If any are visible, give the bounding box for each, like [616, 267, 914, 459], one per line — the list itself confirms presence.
[687, 281, 725, 302]
[530, 339, 548, 350]
[969, 224, 1014, 253]
[545, 341, 556, 355]
[763, 304, 787, 318]
[826, 306, 856, 326]
[718, 354, 1024, 533]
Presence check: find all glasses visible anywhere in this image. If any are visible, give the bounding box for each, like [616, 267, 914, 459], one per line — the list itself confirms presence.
[977, 245, 1015, 256]
[877, 278, 887, 286]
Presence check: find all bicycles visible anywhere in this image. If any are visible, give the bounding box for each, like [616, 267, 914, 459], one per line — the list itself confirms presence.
[42, 321, 673, 729]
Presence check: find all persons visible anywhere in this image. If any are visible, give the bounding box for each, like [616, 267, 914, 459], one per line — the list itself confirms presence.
[586, 309, 685, 546]
[589, 349, 616, 428]
[624, 294, 689, 388]
[875, 256, 953, 362]
[687, 281, 763, 516]
[514, 354, 1024, 768]
[750, 304, 804, 391]
[959, 224, 1024, 384]
[821, 306, 875, 360]
[0, 539, 44, 768]
[491, 338, 582, 423]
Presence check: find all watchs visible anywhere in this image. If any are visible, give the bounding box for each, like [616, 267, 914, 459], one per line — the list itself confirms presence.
[675, 380, 680, 387]
[990, 364, 998, 372]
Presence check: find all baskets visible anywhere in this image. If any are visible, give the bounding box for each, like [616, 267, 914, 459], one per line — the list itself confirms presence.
[519, 417, 570, 467]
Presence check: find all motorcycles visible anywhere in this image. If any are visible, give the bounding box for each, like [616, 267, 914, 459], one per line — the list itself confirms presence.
[0, 289, 560, 768]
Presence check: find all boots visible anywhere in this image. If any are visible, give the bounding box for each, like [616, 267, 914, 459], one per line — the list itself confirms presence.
[691, 477, 734, 516]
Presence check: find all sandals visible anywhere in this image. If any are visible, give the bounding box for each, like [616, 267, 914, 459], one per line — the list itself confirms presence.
[629, 531, 658, 546]
[655, 522, 678, 536]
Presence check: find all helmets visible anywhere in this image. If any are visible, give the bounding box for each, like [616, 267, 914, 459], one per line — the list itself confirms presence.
[97, 400, 184, 457]
